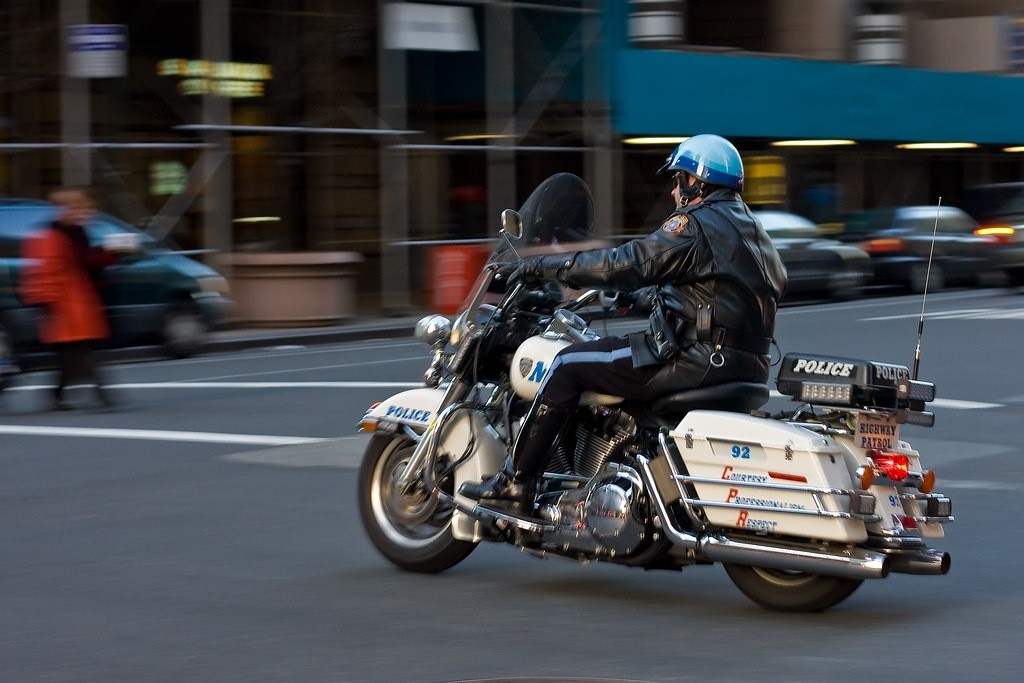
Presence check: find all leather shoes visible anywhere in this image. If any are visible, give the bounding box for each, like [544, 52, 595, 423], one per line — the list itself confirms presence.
[458, 471, 534, 517]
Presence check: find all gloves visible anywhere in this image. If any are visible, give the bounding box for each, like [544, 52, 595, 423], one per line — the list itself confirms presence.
[483, 251, 585, 291]
[614, 285, 656, 316]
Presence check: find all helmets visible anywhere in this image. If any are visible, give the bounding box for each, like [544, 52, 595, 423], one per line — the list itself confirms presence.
[656, 134, 745, 193]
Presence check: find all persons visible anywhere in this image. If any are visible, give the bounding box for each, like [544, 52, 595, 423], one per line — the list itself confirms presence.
[17, 190, 140, 409]
[460, 133, 787, 510]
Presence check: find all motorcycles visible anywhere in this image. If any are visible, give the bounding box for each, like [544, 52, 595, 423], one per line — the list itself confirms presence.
[354, 173, 953, 613]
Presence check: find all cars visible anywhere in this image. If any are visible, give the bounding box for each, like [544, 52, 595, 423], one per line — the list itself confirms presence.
[752, 211, 875, 303]
[854, 206, 1011, 293]
[0, 198, 239, 374]
[973, 204, 1024, 286]
